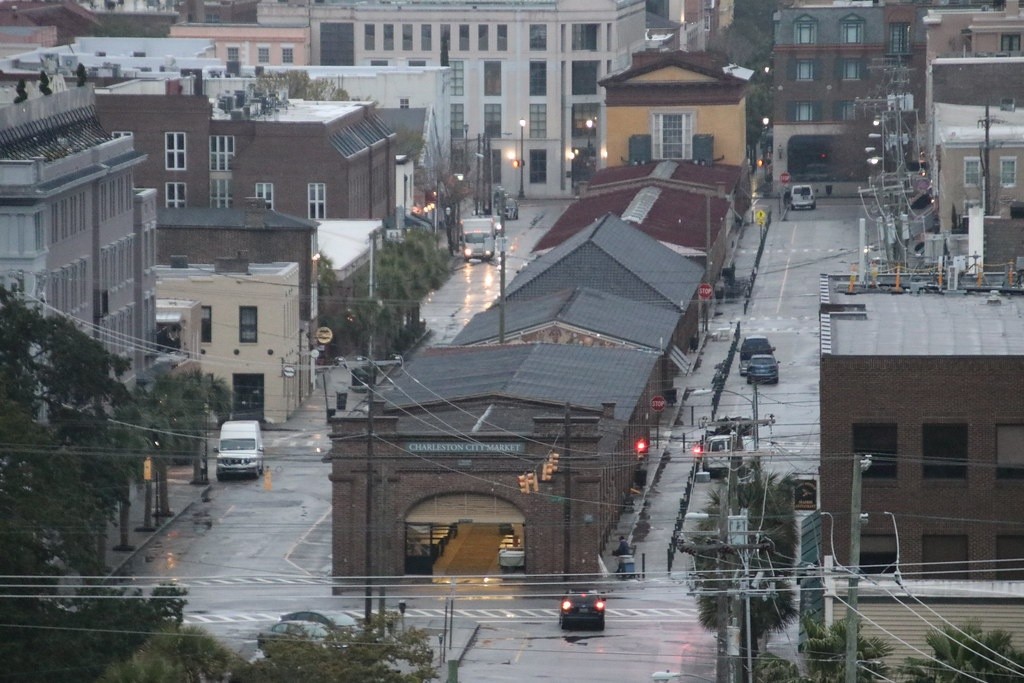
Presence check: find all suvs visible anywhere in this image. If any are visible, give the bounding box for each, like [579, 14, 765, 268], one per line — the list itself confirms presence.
[789, 185, 816, 211]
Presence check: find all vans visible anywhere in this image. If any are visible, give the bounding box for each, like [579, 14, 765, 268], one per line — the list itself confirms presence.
[213, 420, 264, 480]
[736, 337, 776, 376]
[703, 435, 732, 479]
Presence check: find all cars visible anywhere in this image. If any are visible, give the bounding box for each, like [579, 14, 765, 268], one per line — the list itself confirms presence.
[258, 610, 360, 658]
[746, 355, 781, 384]
[497, 198, 520, 220]
[559, 589, 606, 629]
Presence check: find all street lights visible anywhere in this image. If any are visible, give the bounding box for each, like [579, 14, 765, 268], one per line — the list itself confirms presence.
[586, 119, 593, 147]
[761, 116, 769, 181]
[464, 123, 469, 158]
[684, 512, 729, 683]
[519, 118, 526, 198]
[865, 115, 898, 260]
[690, 389, 760, 481]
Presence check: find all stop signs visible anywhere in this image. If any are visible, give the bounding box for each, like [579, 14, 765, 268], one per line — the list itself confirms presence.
[698, 283, 712, 297]
[781, 173, 789, 182]
[651, 396, 665, 412]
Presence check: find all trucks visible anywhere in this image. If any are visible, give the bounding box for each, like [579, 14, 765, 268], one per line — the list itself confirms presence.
[459, 219, 495, 263]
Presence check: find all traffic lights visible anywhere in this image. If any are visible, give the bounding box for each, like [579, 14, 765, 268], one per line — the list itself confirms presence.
[638, 437, 648, 453]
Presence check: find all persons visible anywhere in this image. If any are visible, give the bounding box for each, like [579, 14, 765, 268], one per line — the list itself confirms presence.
[614, 536, 629, 576]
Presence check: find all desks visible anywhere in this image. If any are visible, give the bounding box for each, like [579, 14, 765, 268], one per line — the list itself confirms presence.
[412, 527, 457, 563]
[498, 532, 524, 573]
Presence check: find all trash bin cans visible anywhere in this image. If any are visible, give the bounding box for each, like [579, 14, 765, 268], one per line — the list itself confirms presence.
[335, 390, 348, 410]
[620, 558, 634, 576]
[663, 389, 678, 406]
[633, 469, 648, 490]
[327, 408, 335, 423]
[688, 337, 699, 353]
